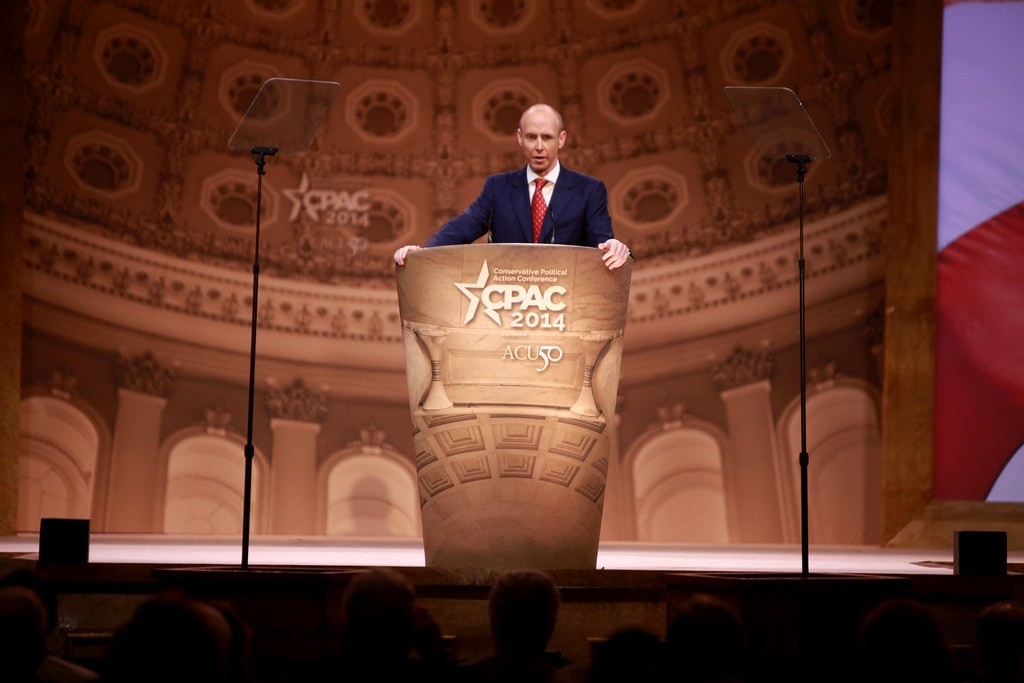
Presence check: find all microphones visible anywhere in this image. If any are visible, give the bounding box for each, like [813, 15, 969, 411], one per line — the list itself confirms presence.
[549, 202, 556, 245]
[488, 208, 492, 244]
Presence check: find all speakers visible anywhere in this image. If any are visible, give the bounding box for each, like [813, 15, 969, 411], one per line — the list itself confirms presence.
[38, 518, 90, 564]
[952, 530, 1008, 576]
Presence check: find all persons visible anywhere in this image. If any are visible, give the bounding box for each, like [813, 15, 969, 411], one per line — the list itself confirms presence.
[0, 566, 1024, 683]
[393, 101, 629, 270]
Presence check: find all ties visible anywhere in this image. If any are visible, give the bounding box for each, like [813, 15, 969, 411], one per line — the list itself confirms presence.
[530, 173, 547, 245]
